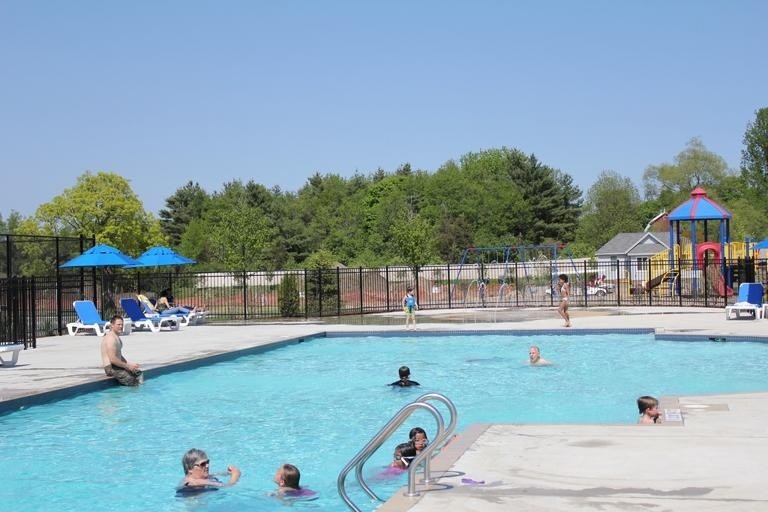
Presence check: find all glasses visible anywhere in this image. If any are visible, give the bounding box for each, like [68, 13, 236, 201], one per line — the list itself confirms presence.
[193, 459, 210, 468]
[393, 453, 401, 461]
[411, 438, 428, 445]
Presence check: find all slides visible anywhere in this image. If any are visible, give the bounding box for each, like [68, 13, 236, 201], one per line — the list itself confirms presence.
[704, 262, 733, 297]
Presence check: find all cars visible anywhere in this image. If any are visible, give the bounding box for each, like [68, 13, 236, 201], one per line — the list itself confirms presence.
[546, 269, 615, 297]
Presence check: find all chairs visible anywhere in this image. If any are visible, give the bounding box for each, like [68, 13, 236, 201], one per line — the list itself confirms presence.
[120, 294, 209, 332]
[0, 344, 24, 366]
[66, 301, 132, 337]
[725, 282, 765, 320]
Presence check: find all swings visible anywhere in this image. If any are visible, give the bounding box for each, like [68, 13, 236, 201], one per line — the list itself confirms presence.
[477, 245, 561, 298]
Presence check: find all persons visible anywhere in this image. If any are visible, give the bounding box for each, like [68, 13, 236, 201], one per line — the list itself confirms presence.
[376, 443, 416, 481]
[401, 285, 421, 331]
[100, 314, 144, 386]
[176, 447, 241, 498]
[154, 290, 198, 314]
[524, 345, 551, 364]
[556, 271, 573, 328]
[386, 365, 420, 387]
[264, 462, 316, 500]
[636, 396, 664, 423]
[165, 288, 195, 310]
[407, 426, 457, 454]
[433, 281, 440, 293]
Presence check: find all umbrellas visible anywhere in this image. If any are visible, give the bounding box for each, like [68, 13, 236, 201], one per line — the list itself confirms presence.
[60, 244, 145, 320]
[120, 245, 199, 293]
